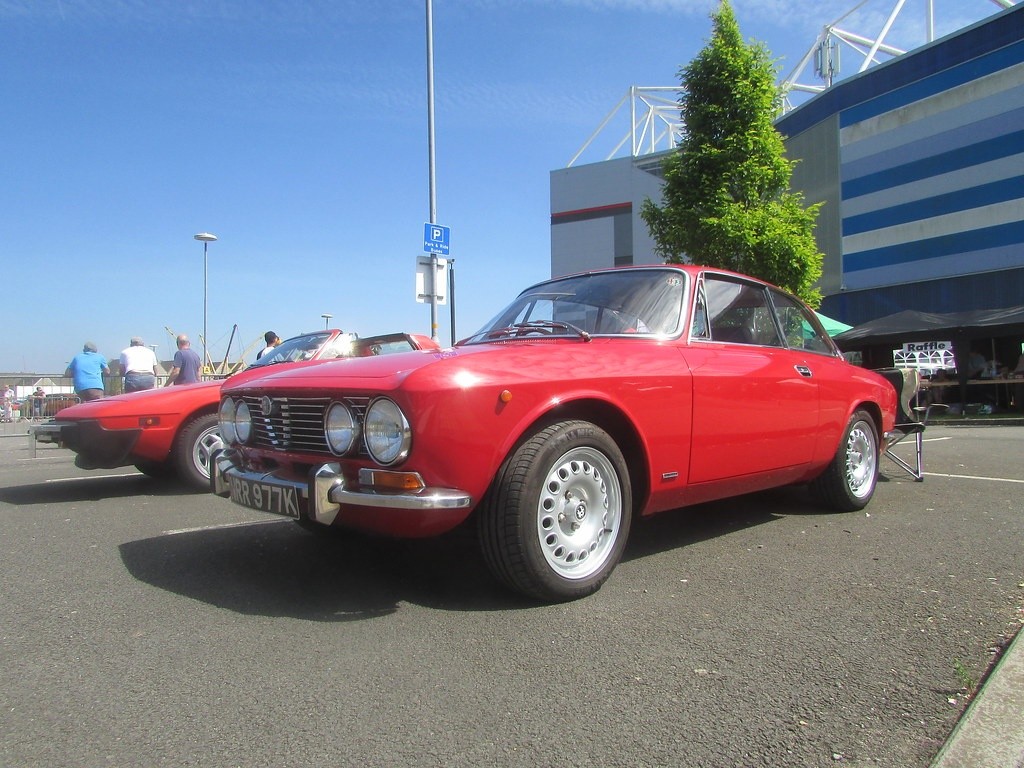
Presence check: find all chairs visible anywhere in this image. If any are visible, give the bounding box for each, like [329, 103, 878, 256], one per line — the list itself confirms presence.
[874, 368, 949, 481]
[707, 326, 759, 344]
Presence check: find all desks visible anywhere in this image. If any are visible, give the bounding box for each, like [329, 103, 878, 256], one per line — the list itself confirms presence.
[928, 378, 1024, 421]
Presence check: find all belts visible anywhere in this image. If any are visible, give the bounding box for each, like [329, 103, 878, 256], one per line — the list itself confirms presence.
[128, 371, 153, 375]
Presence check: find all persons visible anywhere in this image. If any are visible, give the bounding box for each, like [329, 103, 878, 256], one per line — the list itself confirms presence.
[0, 384, 47, 423]
[65, 343, 111, 402]
[257, 330, 279, 360]
[967, 350, 986, 378]
[163, 334, 201, 386]
[119, 336, 158, 393]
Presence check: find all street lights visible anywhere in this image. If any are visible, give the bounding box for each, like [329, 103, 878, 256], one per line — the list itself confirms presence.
[194, 232, 218, 382]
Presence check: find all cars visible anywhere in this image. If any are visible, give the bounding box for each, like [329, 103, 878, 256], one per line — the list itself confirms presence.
[208, 257, 901, 600]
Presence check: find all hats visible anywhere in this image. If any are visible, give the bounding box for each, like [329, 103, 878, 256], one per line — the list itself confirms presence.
[131, 337, 143, 346]
[83, 342, 96, 352]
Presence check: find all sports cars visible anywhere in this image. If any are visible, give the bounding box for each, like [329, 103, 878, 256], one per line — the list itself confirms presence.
[27, 328, 439, 492]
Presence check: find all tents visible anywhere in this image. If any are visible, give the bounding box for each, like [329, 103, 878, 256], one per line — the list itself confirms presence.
[801, 307, 1024, 421]
